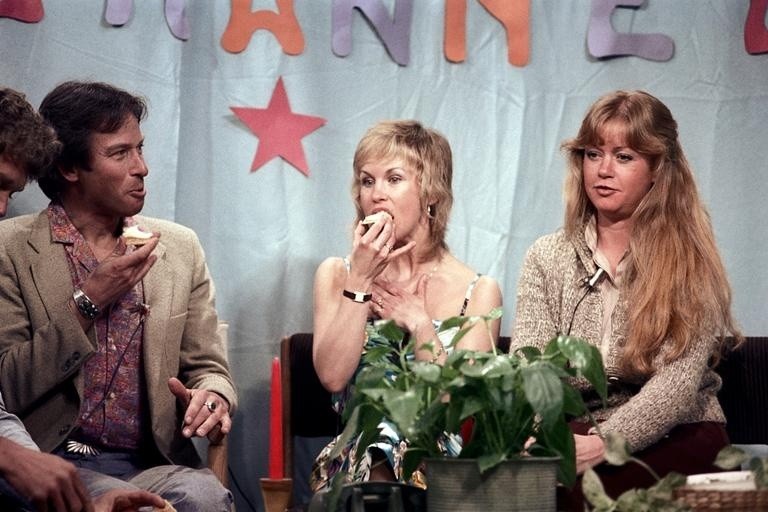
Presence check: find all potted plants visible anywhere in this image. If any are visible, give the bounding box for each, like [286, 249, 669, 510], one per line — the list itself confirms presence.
[328, 306, 607, 512]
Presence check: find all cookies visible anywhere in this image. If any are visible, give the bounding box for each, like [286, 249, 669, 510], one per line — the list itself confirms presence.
[122, 226, 153, 245]
[361, 211, 392, 229]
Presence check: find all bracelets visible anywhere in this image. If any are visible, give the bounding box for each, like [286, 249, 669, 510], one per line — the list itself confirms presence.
[72, 289, 100, 320]
[342, 288, 372, 303]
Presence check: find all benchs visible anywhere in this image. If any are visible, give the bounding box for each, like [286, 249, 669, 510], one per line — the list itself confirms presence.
[258, 332, 768, 512]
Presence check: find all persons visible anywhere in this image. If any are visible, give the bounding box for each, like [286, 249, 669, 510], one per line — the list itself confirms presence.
[500, 90, 741, 510]
[0, 79, 238, 512]
[0, 84, 173, 511]
[306, 119, 504, 511]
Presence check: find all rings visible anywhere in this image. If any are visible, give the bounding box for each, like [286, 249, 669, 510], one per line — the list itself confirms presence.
[377, 297, 383, 306]
[204, 400, 216, 412]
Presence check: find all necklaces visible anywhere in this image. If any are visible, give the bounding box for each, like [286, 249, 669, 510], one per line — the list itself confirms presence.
[385, 257, 441, 293]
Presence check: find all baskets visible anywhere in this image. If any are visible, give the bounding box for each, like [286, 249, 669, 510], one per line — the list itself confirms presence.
[674, 488, 768, 511]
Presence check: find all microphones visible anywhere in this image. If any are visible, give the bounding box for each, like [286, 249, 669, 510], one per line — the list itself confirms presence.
[135, 302, 151, 314]
[580, 267, 605, 290]
